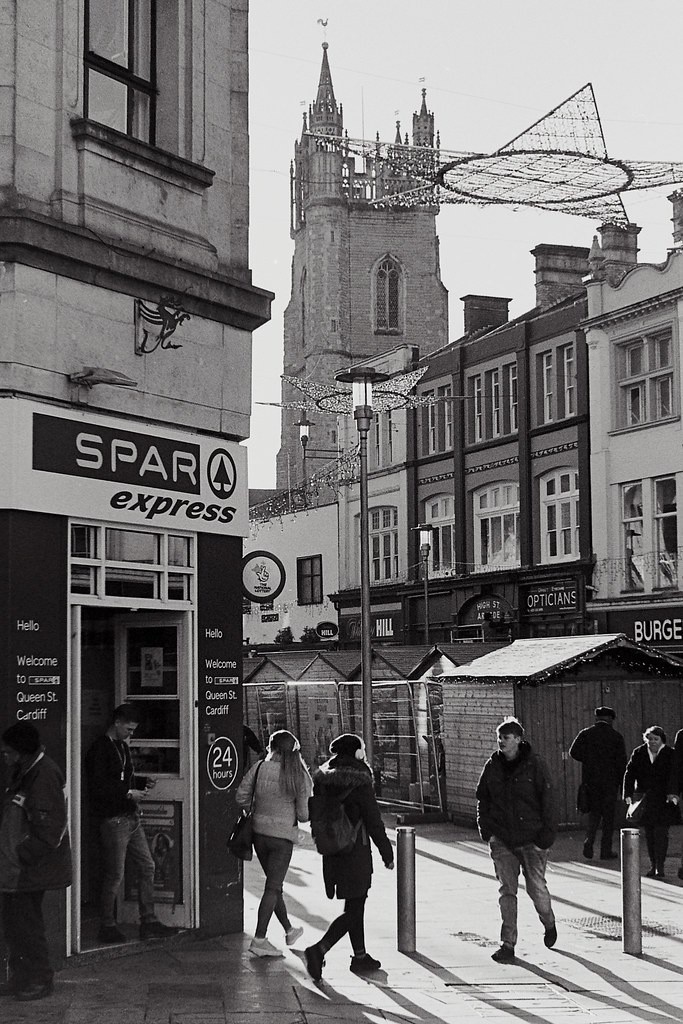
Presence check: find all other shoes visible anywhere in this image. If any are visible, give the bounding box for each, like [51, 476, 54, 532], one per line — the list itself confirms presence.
[583, 845, 593, 859]
[14, 980, 53, 1000]
[98, 926, 130, 944]
[655, 863, 664, 877]
[601, 852, 617, 860]
[544, 927, 557, 947]
[0, 981, 21, 995]
[646, 868, 655, 876]
[491, 947, 515, 959]
[140, 922, 178, 940]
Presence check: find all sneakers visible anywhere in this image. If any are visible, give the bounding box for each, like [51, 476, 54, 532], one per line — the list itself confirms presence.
[285, 927, 303, 945]
[249, 938, 282, 957]
[306, 945, 323, 980]
[350, 953, 381, 972]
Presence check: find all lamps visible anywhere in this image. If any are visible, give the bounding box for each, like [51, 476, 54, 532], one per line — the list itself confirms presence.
[68, 366, 138, 391]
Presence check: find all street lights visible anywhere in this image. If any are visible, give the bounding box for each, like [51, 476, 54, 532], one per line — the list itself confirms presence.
[292, 419, 317, 492]
[334, 365, 392, 767]
[409, 523, 440, 646]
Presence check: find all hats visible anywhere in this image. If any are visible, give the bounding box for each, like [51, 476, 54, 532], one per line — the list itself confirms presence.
[2, 721, 39, 753]
[330, 734, 361, 755]
[595, 706, 616, 720]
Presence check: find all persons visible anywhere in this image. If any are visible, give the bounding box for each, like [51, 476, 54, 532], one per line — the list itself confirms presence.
[475, 716, 557, 963]
[235, 730, 314, 957]
[569, 707, 627, 860]
[86, 702, 180, 945]
[304, 733, 394, 980]
[243, 725, 267, 784]
[0, 719, 73, 1001]
[624, 727, 683, 879]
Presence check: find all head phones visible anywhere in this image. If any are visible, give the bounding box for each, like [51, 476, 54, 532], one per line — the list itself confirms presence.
[355, 734, 365, 761]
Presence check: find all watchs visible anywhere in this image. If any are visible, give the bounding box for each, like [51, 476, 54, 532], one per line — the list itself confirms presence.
[126, 790, 133, 800]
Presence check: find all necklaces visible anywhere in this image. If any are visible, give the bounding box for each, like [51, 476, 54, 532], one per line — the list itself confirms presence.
[107, 733, 127, 781]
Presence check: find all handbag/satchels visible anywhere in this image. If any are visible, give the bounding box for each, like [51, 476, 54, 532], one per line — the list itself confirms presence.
[227, 809, 254, 861]
[576, 785, 589, 813]
[626, 794, 647, 823]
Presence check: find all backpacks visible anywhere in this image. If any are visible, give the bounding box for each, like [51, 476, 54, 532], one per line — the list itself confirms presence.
[308, 784, 363, 855]
